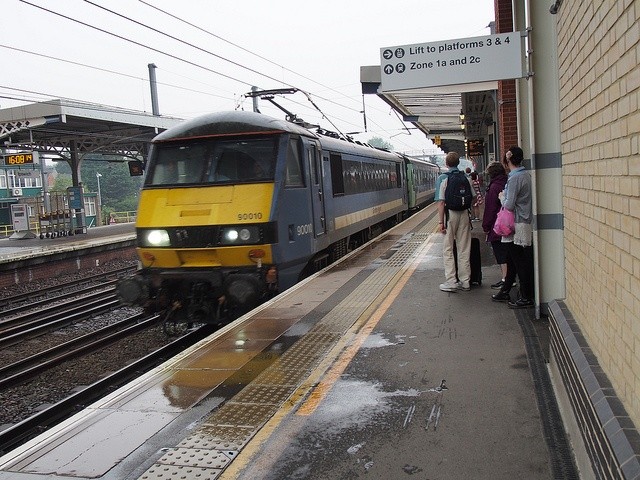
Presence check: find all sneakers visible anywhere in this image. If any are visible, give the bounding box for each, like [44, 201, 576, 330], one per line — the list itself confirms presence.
[508, 297, 532, 307]
[511, 281, 518, 287]
[455, 282, 470, 292]
[475, 217, 481, 222]
[470, 218, 475, 221]
[491, 290, 510, 302]
[491, 280, 506, 289]
[440, 283, 456, 292]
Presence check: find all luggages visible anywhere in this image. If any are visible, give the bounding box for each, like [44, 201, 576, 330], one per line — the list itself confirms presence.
[453, 238, 482, 287]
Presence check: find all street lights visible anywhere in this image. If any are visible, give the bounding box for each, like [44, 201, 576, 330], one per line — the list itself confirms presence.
[96, 172, 102, 206]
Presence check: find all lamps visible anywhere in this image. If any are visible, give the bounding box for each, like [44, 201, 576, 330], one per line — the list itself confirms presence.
[464, 136, 468, 143]
[459, 109, 465, 120]
[460, 121, 466, 130]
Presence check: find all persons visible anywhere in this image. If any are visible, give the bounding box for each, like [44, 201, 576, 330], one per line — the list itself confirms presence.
[250, 160, 271, 179]
[481, 161, 518, 290]
[465, 168, 471, 177]
[491, 145, 535, 308]
[433, 152, 477, 293]
[469, 173, 484, 222]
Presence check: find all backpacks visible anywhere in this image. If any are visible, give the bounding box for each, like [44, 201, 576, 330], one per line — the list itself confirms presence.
[441, 170, 472, 211]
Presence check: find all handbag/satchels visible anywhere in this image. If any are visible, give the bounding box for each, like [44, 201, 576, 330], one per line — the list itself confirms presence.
[493, 206, 516, 237]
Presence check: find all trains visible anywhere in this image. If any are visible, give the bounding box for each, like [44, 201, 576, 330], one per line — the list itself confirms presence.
[116, 111, 448, 335]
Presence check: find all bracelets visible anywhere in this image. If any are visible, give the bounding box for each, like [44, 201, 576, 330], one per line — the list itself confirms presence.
[439, 222, 444, 224]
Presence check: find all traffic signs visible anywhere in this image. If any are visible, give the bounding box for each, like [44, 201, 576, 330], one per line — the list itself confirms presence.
[380, 31, 523, 92]
